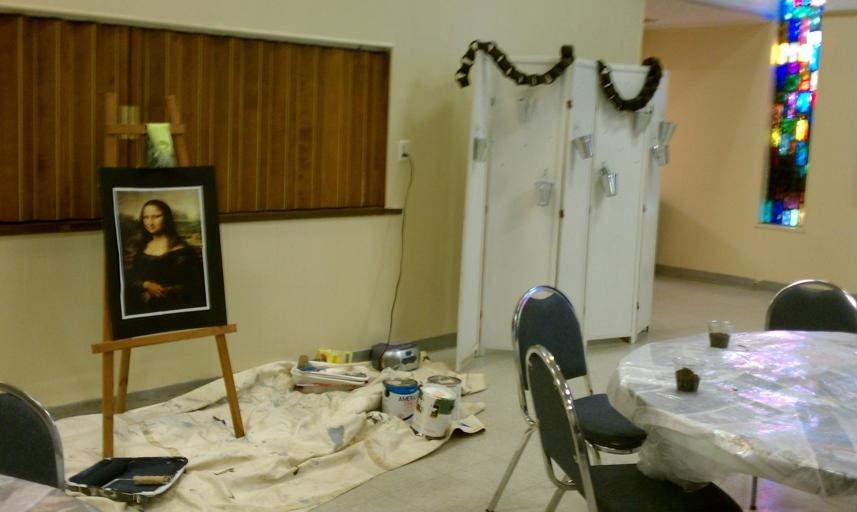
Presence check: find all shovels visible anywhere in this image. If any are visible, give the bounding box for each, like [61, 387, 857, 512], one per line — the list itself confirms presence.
[100, 476, 170, 487]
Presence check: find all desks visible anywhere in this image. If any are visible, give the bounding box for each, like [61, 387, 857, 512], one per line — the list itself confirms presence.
[609, 329, 857, 507]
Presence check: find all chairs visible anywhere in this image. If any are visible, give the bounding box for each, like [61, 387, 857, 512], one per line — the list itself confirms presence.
[485, 284, 655, 512]
[524, 344, 746, 510]
[0, 377, 67, 492]
[764, 279, 857, 333]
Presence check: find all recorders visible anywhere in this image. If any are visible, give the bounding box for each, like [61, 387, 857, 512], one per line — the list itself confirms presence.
[370, 342, 420, 372]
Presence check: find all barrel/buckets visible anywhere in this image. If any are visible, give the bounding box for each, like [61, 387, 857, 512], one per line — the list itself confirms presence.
[412, 383, 457, 439]
[427, 376, 462, 421]
[516, 85, 678, 205]
[381, 378, 418, 422]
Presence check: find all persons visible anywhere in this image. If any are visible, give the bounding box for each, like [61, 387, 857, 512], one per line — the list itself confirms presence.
[123, 200, 205, 309]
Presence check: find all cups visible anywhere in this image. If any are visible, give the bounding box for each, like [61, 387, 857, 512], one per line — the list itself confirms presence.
[600, 173, 617, 197]
[572, 134, 594, 162]
[633, 108, 654, 133]
[708, 319, 735, 350]
[672, 357, 708, 392]
[650, 121, 679, 167]
[474, 137, 491, 163]
[518, 97, 535, 124]
[534, 181, 553, 207]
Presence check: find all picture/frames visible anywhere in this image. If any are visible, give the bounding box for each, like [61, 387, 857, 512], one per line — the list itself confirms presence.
[97, 163, 227, 344]
